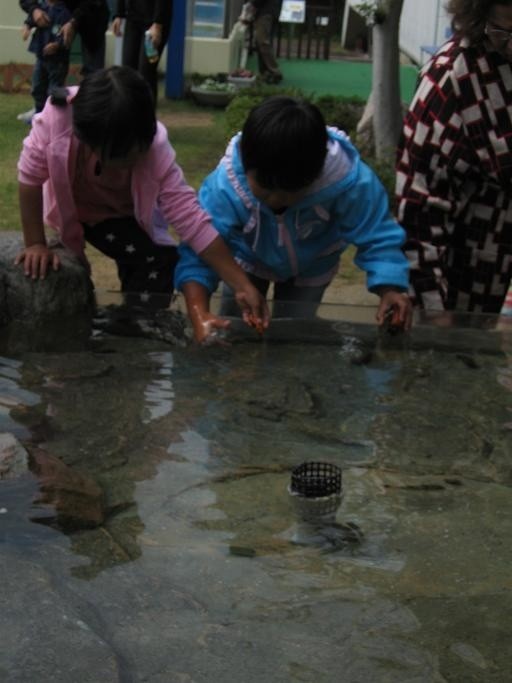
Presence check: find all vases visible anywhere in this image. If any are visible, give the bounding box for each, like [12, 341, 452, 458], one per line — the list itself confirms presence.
[227, 75, 256, 89]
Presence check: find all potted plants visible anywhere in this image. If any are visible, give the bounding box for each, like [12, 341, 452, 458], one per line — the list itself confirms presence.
[189, 72, 236, 108]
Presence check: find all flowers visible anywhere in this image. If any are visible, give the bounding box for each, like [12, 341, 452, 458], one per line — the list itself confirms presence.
[231, 68, 252, 77]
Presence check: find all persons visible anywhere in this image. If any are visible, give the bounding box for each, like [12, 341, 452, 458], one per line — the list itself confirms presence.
[248, 0, 283, 85]
[19, 0, 111, 80]
[395, 0, 512, 329]
[228, 2, 255, 78]
[172, 89, 414, 346]
[15, 65, 271, 334]
[16, 1, 72, 122]
[111, 1, 174, 108]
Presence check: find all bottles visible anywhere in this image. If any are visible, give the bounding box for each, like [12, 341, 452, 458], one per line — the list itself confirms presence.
[144, 31, 159, 64]
